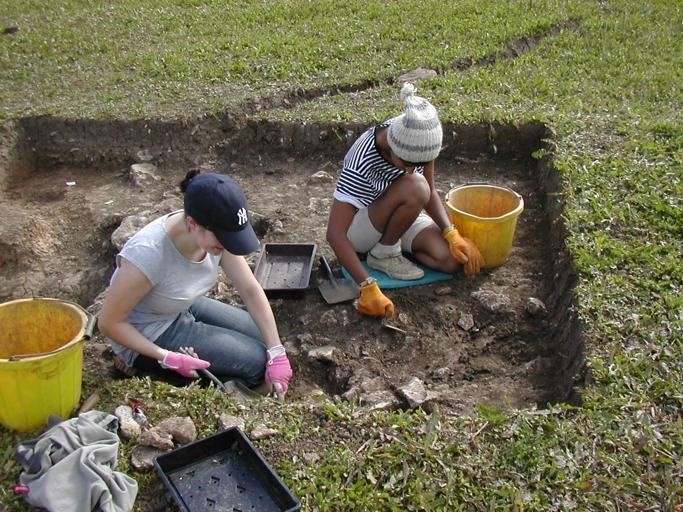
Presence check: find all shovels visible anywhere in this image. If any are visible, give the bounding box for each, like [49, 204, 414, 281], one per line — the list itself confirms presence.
[381, 318, 408, 334]
[175, 346, 264, 401]
[46, 390, 100, 432]
[316, 255, 361, 305]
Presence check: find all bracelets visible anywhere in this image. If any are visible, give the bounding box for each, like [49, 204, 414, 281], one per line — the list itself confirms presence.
[358, 276, 377, 287]
[441, 224, 454, 236]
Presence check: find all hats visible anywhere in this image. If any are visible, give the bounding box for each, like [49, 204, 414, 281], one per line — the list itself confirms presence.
[386, 82, 444, 164]
[184, 173, 260, 256]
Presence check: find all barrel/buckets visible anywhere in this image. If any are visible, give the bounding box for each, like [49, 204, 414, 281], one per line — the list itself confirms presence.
[444, 182, 524, 270]
[0, 293, 97, 434]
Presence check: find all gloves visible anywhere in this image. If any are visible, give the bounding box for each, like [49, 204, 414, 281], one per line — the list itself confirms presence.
[157, 347, 211, 378]
[264, 345, 292, 404]
[443, 227, 486, 275]
[356, 281, 395, 320]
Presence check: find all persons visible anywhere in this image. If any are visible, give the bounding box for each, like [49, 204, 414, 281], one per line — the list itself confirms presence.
[325, 81, 486, 320]
[95, 168, 293, 401]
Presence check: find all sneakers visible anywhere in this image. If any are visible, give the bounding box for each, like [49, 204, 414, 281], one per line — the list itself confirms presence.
[366, 250, 425, 280]
